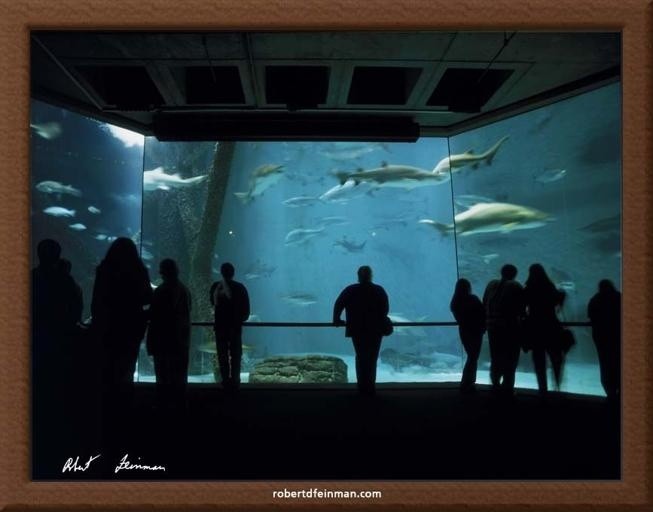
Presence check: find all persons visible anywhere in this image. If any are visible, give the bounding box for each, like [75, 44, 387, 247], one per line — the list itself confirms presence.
[209, 264, 249, 385]
[521, 266, 565, 395]
[148, 260, 191, 400]
[88, 237, 153, 383]
[333, 266, 392, 399]
[482, 265, 527, 393]
[451, 280, 486, 393]
[587, 281, 619, 399]
[33, 239, 83, 381]
